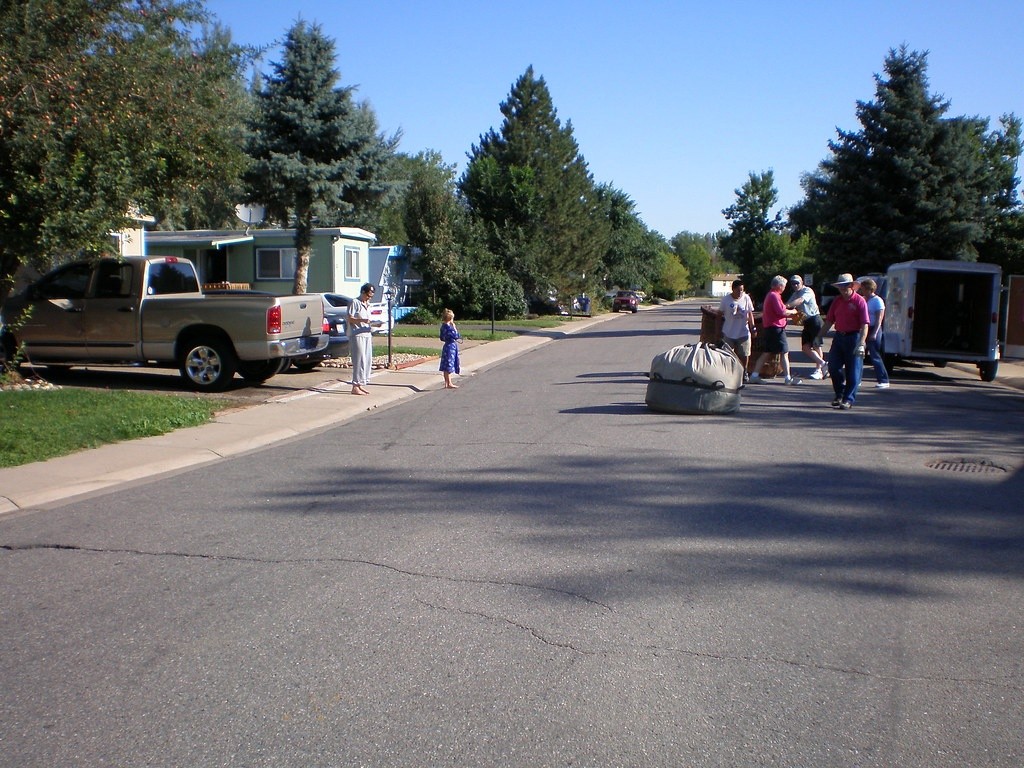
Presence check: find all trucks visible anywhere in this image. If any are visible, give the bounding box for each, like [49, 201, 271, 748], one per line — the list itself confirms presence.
[856, 257, 1024, 384]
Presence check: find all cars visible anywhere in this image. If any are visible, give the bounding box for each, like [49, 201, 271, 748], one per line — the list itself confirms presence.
[303, 291, 395, 342]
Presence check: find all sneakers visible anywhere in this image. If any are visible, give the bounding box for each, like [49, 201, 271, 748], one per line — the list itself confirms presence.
[750, 375, 767, 384]
[743, 372, 749, 384]
[832, 397, 853, 410]
[809, 362, 830, 380]
[785, 376, 803, 384]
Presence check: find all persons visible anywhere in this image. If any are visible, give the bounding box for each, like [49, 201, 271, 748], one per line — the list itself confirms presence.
[345, 283, 376, 394]
[859, 279, 890, 388]
[749, 275, 804, 385]
[714, 280, 758, 383]
[783, 275, 830, 380]
[439, 308, 463, 388]
[813, 273, 871, 409]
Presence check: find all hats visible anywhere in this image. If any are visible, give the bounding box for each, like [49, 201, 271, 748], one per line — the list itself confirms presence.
[790, 275, 802, 284]
[830, 274, 860, 289]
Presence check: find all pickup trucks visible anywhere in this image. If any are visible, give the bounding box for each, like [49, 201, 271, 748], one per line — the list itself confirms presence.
[0, 255, 331, 393]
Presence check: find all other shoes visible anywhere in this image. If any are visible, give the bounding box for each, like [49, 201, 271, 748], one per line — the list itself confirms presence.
[351, 386, 369, 395]
[446, 382, 459, 389]
[875, 382, 890, 388]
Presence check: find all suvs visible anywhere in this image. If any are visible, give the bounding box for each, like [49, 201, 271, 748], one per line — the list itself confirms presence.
[602, 288, 644, 313]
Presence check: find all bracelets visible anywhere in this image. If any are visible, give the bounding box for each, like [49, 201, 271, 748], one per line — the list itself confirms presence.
[752, 326, 756, 333]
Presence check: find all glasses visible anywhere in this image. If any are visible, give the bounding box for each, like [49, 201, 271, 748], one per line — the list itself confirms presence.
[366, 293, 373, 298]
[791, 282, 799, 285]
